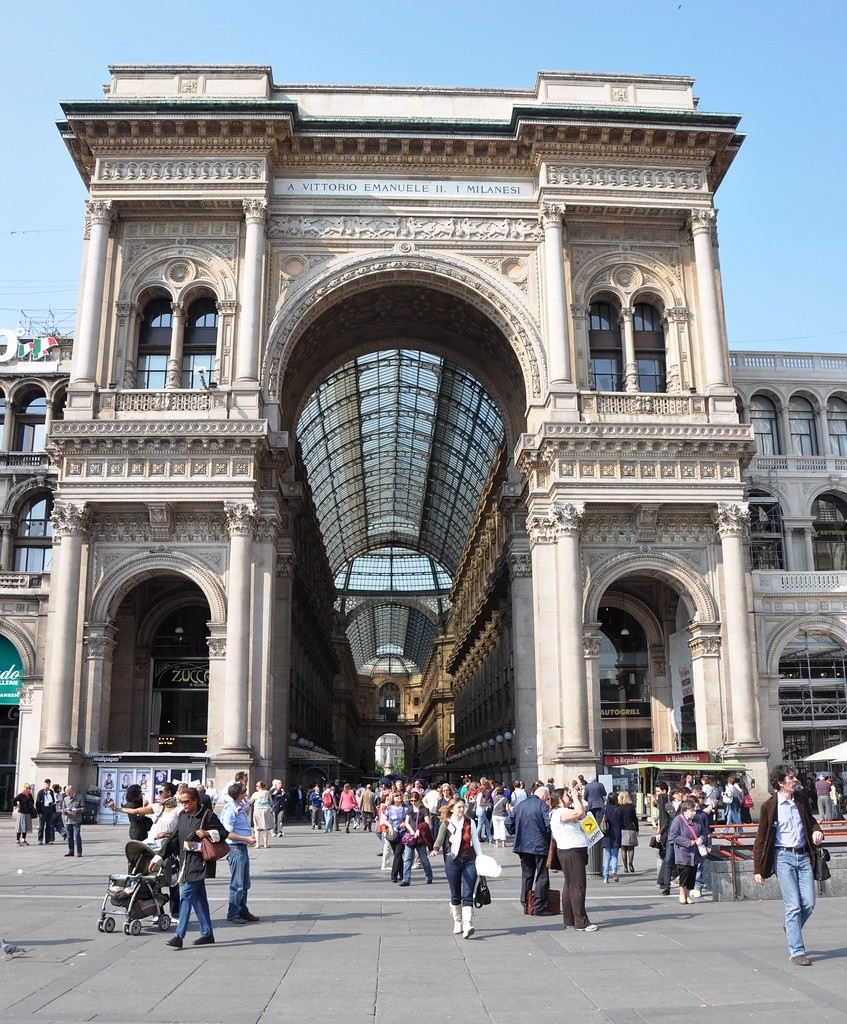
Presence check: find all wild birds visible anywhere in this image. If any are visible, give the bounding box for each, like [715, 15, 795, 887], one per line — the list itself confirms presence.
[0, 938, 26, 959]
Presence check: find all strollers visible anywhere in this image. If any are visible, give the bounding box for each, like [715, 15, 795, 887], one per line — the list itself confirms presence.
[97, 832, 181, 936]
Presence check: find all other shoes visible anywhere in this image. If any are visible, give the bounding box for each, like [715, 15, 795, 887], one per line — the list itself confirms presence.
[791, 954, 811, 966]
[603, 864, 703, 905]
[254, 832, 284, 850]
[576, 925, 597, 931]
[151, 913, 259, 949]
[14, 832, 82, 858]
[377, 850, 439, 886]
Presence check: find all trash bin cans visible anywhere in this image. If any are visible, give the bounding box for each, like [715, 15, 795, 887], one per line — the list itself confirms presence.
[586, 840, 604, 879]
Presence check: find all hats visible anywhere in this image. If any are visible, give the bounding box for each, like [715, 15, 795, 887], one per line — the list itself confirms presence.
[818, 773, 825, 777]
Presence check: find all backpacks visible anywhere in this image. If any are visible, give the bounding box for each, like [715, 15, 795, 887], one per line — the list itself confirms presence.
[323, 791, 333, 807]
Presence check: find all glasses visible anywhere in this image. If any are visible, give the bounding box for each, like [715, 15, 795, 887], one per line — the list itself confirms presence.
[179, 799, 193, 805]
[159, 790, 164, 794]
[393, 794, 401, 797]
[408, 799, 418, 802]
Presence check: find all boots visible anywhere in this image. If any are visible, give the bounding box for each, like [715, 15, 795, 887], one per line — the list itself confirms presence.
[462, 906, 474, 939]
[451, 902, 461, 934]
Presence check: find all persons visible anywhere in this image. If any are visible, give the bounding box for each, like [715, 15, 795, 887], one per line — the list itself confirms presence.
[752, 764, 824, 966]
[794, 770, 837, 822]
[104, 774, 114, 789]
[12, 783, 34, 846]
[120, 793, 128, 807]
[155, 785, 162, 800]
[504, 775, 639, 932]
[459, 777, 511, 848]
[103, 793, 114, 808]
[156, 770, 167, 785]
[51, 784, 67, 840]
[428, 797, 483, 939]
[139, 773, 148, 789]
[290, 779, 459, 887]
[249, 781, 272, 849]
[269, 778, 289, 837]
[652, 773, 752, 905]
[121, 774, 131, 789]
[61, 786, 86, 856]
[108, 771, 258, 948]
[36, 778, 55, 844]
[141, 793, 149, 807]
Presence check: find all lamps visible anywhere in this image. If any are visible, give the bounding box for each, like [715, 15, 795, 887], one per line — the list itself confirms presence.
[175, 610, 184, 633]
[304, 740, 329, 755]
[446, 744, 482, 763]
[504, 731, 514, 749]
[496, 734, 504, 751]
[489, 739, 496, 752]
[482, 742, 488, 753]
[298, 738, 304, 747]
[621, 593, 629, 635]
[290, 732, 297, 740]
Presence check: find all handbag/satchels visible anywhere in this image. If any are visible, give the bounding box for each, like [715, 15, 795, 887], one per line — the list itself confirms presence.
[201, 808, 231, 860]
[812, 851, 831, 881]
[402, 832, 417, 847]
[722, 793, 733, 804]
[698, 844, 707, 856]
[528, 890, 561, 916]
[474, 875, 491, 908]
[578, 811, 604, 849]
[744, 795, 754, 808]
[599, 817, 610, 834]
[386, 829, 400, 841]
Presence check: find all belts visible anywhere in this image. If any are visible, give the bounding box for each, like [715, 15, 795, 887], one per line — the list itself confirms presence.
[776, 847, 807, 853]
